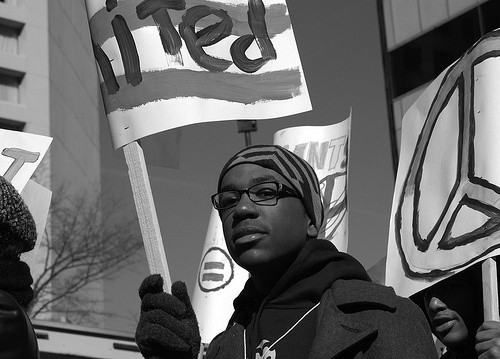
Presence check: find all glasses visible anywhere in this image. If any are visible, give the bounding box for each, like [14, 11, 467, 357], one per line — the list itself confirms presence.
[211, 182, 298, 210]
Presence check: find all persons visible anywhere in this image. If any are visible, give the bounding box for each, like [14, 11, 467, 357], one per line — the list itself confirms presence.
[420, 269, 500, 359]
[0, 176, 41, 359]
[136, 145, 437, 359]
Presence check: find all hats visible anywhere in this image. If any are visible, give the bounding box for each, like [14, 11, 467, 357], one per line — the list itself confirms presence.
[0, 176, 37, 254]
[218, 144, 324, 232]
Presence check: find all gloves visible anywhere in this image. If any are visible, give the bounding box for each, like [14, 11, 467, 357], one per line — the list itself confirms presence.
[136, 274, 201, 359]
